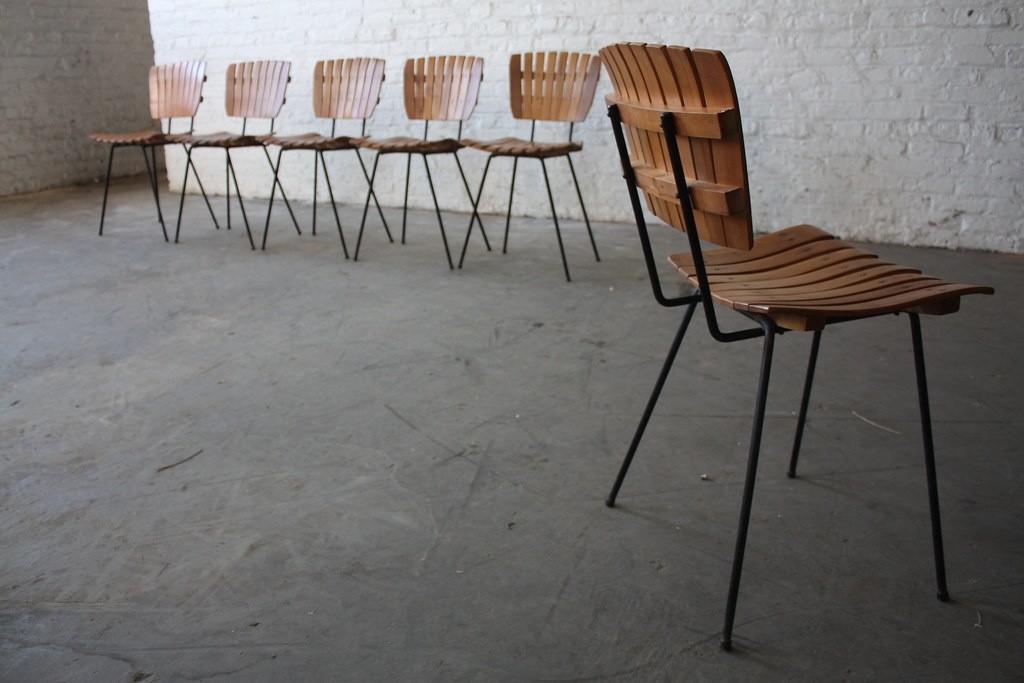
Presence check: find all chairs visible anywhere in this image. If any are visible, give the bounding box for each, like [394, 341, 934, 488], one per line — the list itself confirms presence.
[598, 39, 995, 650]
[175, 59, 303, 244]
[457, 52, 601, 283]
[89, 60, 220, 242]
[254, 57, 393, 260]
[350, 56, 492, 271]
